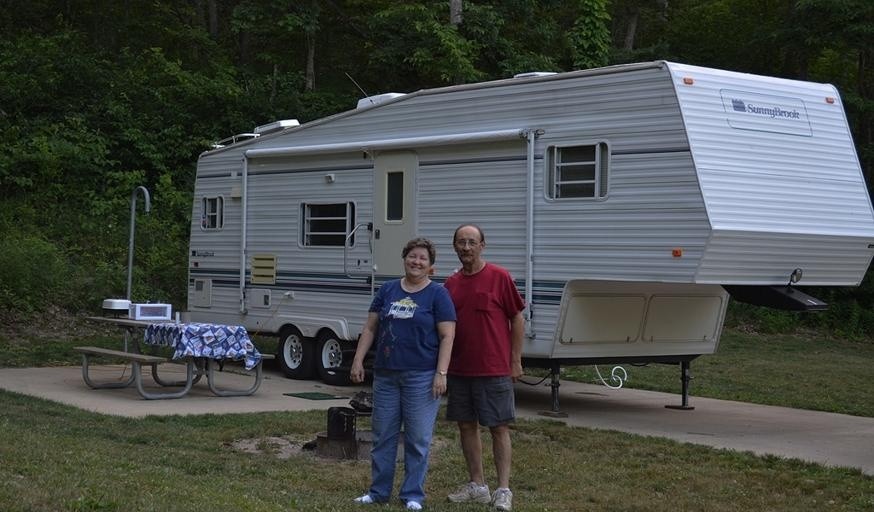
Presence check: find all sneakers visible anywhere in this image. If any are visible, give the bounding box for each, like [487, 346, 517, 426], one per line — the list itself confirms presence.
[406, 500, 422, 511]
[447, 481, 491, 504]
[353, 494, 373, 504]
[490, 488, 512, 512]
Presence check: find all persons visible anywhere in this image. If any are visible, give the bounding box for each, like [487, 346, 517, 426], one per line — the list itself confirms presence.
[348, 236, 458, 511]
[443, 224, 528, 511]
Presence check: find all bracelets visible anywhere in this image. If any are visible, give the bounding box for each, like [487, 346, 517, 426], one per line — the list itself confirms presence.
[435, 369, 448, 376]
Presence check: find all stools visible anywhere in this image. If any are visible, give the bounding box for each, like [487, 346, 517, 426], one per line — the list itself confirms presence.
[251, 353, 275, 395]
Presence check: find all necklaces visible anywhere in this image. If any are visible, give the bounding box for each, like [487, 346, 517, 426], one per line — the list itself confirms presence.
[463, 259, 484, 275]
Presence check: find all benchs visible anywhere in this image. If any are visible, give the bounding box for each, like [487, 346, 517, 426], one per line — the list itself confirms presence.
[74, 343, 169, 400]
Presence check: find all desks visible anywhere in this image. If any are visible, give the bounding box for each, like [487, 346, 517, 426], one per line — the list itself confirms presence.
[81, 316, 263, 400]
